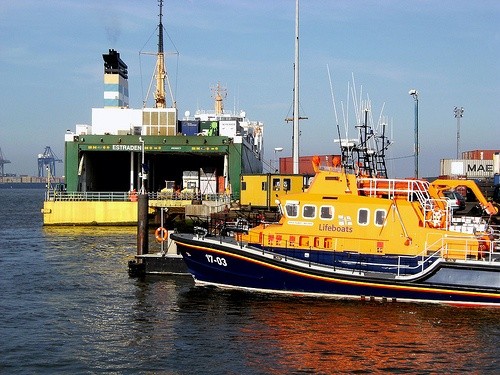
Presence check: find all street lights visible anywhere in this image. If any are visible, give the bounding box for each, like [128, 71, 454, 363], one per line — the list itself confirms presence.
[454, 106, 464, 158]
[408, 89, 419, 179]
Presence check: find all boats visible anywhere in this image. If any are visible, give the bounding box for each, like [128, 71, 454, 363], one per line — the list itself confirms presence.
[129, 0, 500, 310]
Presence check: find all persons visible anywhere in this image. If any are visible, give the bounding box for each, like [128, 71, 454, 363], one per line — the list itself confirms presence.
[224, 205, 230, 217]
[176, 188, 201, 200]
[248, 203, 252, 210]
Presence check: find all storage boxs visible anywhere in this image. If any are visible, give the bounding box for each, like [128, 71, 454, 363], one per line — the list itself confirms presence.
[141, 107, 245, 138]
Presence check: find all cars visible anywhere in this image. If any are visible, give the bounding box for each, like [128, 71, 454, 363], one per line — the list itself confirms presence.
[442, 191, 466, 214]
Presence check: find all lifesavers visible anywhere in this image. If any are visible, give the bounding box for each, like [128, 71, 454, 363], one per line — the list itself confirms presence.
[155, 227, 167, 241]
[427, 208, 446, 228]
